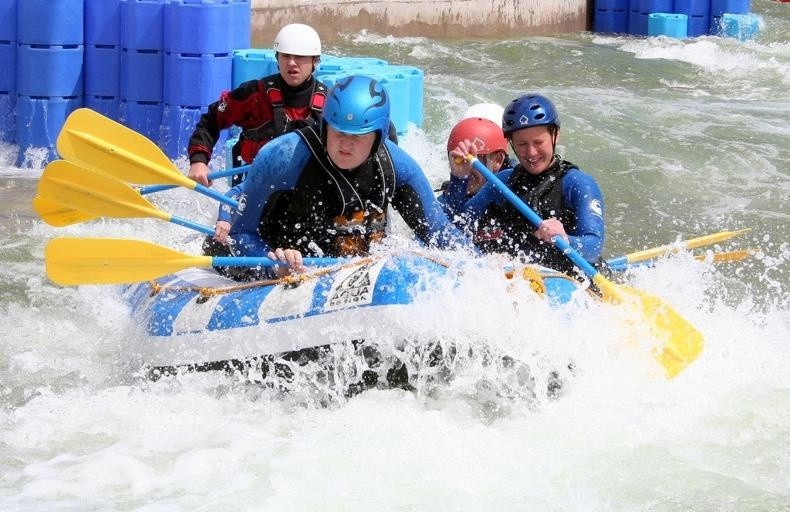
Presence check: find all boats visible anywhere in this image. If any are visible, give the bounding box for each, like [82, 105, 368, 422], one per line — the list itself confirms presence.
[108, 242, 588, 402]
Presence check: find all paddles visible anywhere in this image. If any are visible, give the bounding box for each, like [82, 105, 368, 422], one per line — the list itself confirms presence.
[55, 107, 246, 215]
[32, 162, 257, 228]
[37, 159, 239, 248]
[610, 249, 751, 271]
[43, 237, 360, 285]
[454, 152, 704, 381]
[605, 228, 754, 266]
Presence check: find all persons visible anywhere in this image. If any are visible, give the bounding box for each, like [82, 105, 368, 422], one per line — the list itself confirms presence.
[441, 94, 604, 296]
[227, 75, 484, 281]
[187, 24, 332, 190]
[214, 181, 246, 245]
[437, 118, 520, 208]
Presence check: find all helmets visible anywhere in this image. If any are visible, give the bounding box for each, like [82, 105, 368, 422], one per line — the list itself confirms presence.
[447, 93, 560, 155]
[320, 76, 389, 152]
[274, 23, 322, 56]
